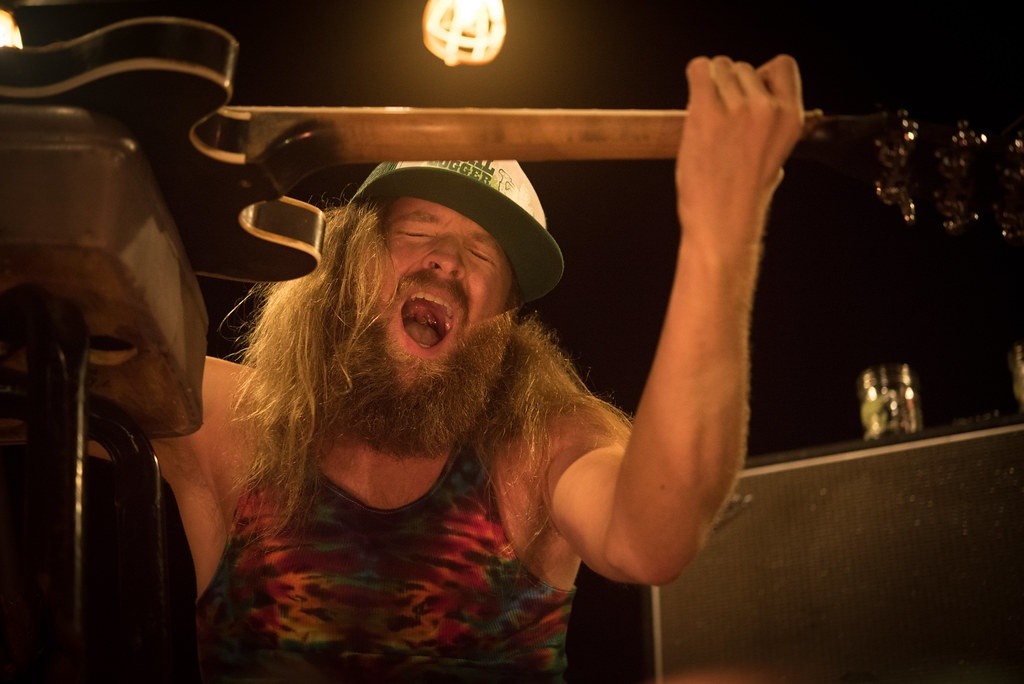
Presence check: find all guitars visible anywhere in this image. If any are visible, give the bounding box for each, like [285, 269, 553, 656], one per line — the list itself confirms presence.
[1, 12, 1024, 288]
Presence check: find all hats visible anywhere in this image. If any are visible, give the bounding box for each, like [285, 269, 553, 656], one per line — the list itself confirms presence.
[340, 158, 566, 302]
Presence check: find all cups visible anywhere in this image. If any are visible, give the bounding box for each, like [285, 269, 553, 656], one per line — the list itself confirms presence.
[858, 365, 922, 441]
[1008, 343, 1024, 414]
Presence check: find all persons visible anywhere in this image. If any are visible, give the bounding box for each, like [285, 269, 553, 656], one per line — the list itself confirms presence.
[152, 53, 803, 683]
